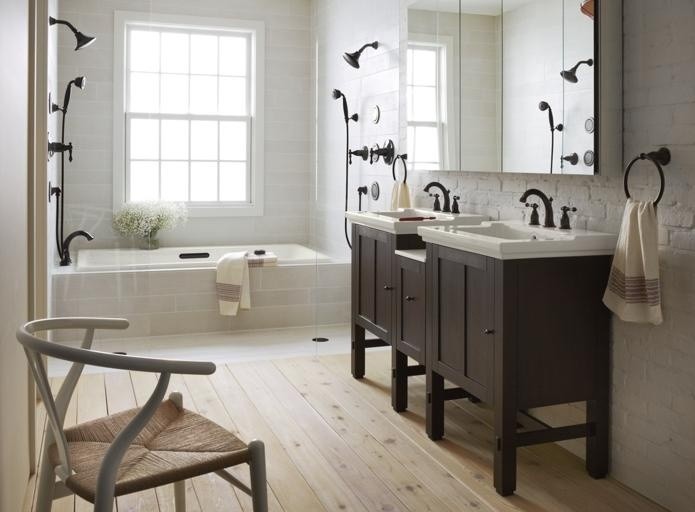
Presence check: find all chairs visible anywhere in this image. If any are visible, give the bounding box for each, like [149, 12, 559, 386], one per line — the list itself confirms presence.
[16, 318, 268, 511]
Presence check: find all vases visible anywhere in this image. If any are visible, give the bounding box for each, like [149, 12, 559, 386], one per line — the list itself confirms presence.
[139, 231, 160, 251]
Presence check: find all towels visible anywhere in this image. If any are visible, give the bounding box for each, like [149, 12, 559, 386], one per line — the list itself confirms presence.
[216, 250, 278, 315]
[391, 183, 411, 210]
[601, 199, 664, 328]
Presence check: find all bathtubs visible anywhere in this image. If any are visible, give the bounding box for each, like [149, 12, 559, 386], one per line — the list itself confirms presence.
[73, 242, 331, 272]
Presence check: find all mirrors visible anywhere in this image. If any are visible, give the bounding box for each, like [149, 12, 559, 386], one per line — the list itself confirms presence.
[399, 1, 600, 176]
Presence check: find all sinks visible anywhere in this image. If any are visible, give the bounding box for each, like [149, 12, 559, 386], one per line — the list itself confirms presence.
[416, 219, 619, 256]
[345, 206, 492, 233]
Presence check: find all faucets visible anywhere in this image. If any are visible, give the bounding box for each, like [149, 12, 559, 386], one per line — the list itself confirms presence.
[424, 180, 454, 214]
[517, 188, 557, 224]
[60, 229, 93, 260]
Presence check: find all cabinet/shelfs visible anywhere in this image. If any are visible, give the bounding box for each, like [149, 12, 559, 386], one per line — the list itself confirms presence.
[351, 224, 615, 496]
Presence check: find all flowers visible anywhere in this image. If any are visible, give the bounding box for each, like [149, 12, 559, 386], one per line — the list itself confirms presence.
[109, 196, 188, 247]
